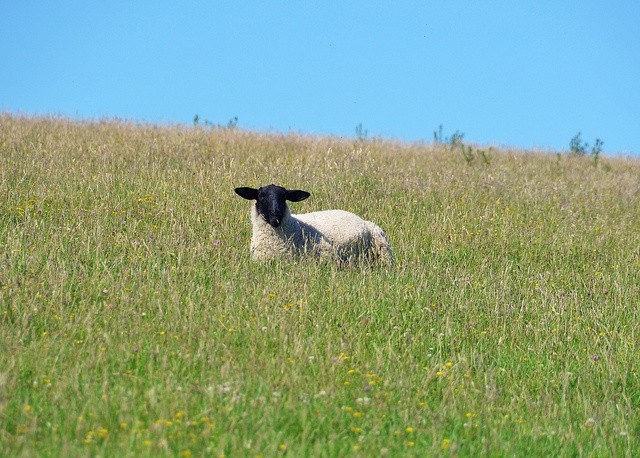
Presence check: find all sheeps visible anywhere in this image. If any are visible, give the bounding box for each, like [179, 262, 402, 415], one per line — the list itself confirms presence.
[234, 183, 392, 271]
